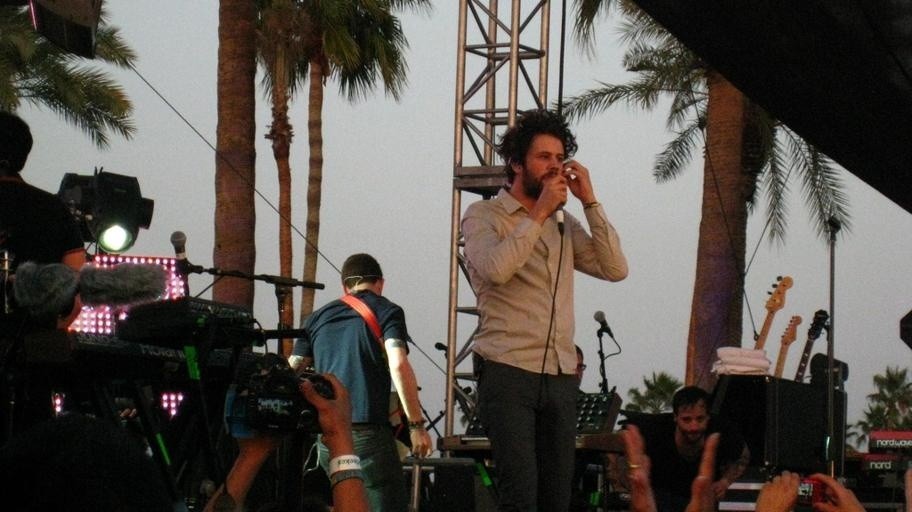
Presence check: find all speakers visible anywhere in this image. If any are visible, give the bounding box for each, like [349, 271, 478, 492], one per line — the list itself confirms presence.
[707, 374, 846, 483]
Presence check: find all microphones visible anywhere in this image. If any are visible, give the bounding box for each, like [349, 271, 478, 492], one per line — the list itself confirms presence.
[171, 231, 190, 294]
[554, 204, 566, 235]
[463, 386, 472, 394]
[593, 310, 614, 338]
[435, 342, 447, 351]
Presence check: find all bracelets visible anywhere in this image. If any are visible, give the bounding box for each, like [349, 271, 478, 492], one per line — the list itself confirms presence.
[582, 202, 601, 209]
[408, 417, 426, 430]
[330, 469, 365, 489]
[328, 453, 363, 479]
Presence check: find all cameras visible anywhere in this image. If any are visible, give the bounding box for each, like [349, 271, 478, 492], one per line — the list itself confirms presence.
[237, 358, 336, 434]
[795, 477, 826, 506]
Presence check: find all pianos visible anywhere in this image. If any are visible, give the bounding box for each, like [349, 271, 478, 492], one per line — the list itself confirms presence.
[438, 433, 625, 457]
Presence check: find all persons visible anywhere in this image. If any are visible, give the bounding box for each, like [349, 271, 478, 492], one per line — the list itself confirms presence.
[617, 420, 723, 512]
[196, 368, 374, 511]
[457, 106, 629, 512]
[1, 110, 87, 333]
[645, 384, 753, 512]
[285, 254, 432, 512]
[574, 343, 587, 389]
[753, 470, 868, 511]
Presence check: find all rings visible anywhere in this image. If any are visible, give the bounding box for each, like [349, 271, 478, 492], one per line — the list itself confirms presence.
[628, 461, 644, 470]
